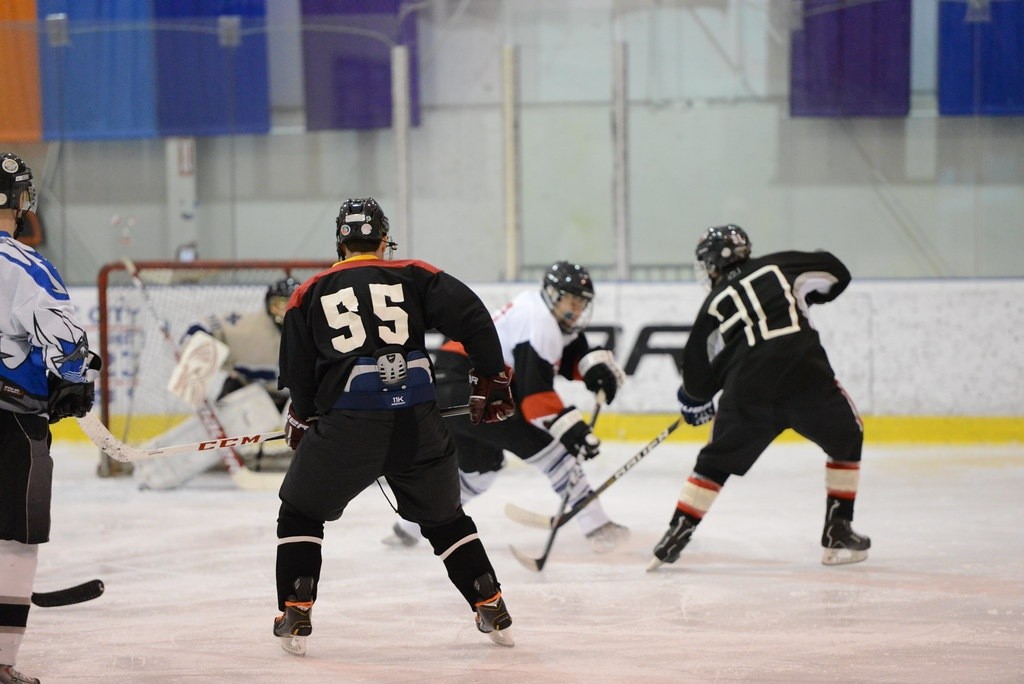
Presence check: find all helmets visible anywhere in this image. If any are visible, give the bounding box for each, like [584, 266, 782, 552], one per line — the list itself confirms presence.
[0, 151, 34, 210]
[695, 223, 751, 288]
[542, 261, 594, 335]
[335, 197, 389, 245]
[264, 277, 300, 327]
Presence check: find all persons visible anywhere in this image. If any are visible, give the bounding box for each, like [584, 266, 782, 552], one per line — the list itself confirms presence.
[380, 260, 637, 550]
[648, 224, 871, 569]
[273, 197, 515, 657]
[186, 276, 300, 473]
[0, 151, 103, 684]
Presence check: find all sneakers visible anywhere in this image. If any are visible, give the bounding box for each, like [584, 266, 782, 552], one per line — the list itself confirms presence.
[472, 572, 515, 646]
[382, 521, 415, 548]
[823, 496, 872, 565]
[0, 665, 40, 684]
[586, 521, 629, 552]
[645, 509, 702, 572]
[274, 574, 315, 654]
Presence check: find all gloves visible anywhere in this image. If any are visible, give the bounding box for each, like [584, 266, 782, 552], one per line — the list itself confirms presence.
[582, 361, 616, 404]
[46, 350, 103, 424]
[284, 398, 311, 449]
[547, 403, 599, 460]
[678, 385, 714, 426]
[468, 364, 517, 424]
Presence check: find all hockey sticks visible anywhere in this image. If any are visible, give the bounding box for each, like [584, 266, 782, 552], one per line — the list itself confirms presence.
[502, 416, 686, 530]
[506, 388, 608, 573]
[75, 411, 505, 465]
[30, 576, 108, 611]
[119, 254, 286, 488]
